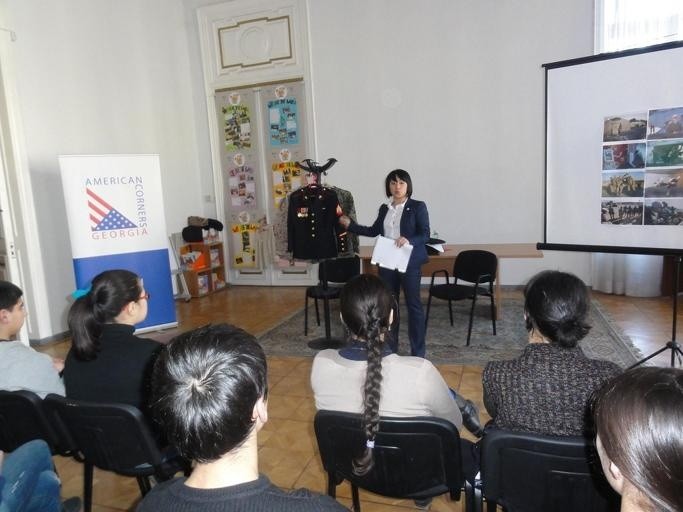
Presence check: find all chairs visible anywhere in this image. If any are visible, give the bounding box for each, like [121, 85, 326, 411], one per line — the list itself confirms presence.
[304, 255, 363, 337]
[473, 431, 621, 511]
[0, 389, 71, 457]
[425, 250, 498, 347]
[314, 409, 473, 512]
[47, 398, 188, 511]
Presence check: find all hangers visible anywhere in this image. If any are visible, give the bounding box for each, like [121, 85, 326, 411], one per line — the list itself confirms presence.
[304, 173, 322, 190]
[322, 175, 335, 189]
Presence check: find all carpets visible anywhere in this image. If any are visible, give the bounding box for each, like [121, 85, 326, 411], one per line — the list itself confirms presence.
[257, 285, 651, 368]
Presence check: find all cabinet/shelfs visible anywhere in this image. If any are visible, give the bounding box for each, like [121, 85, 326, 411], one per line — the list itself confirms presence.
[179, 239, 228, 299]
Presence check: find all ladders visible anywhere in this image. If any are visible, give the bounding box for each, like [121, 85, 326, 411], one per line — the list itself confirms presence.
[168, 234, 192, 303]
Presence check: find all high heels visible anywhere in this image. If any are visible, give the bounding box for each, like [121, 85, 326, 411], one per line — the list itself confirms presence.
[460, 399, 484, 439]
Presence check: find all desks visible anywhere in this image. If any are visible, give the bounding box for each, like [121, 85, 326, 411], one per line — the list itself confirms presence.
[355, 242, 544, 320]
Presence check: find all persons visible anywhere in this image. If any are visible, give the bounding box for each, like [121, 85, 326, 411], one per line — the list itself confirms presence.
[0, 281, 67, 400]
[63, 270, 184, 468]
[135, 323, 350, 512]
[311, 275, 482, 509]
[609, 174, 639, 199]
[340, 170, 430, 356]
[594, 367, 682, 511]
[660, 174, 680, 196]
[0, 440, 82, 511]
[484, 270, 624, 444]
[601, 203, 642, 221]
